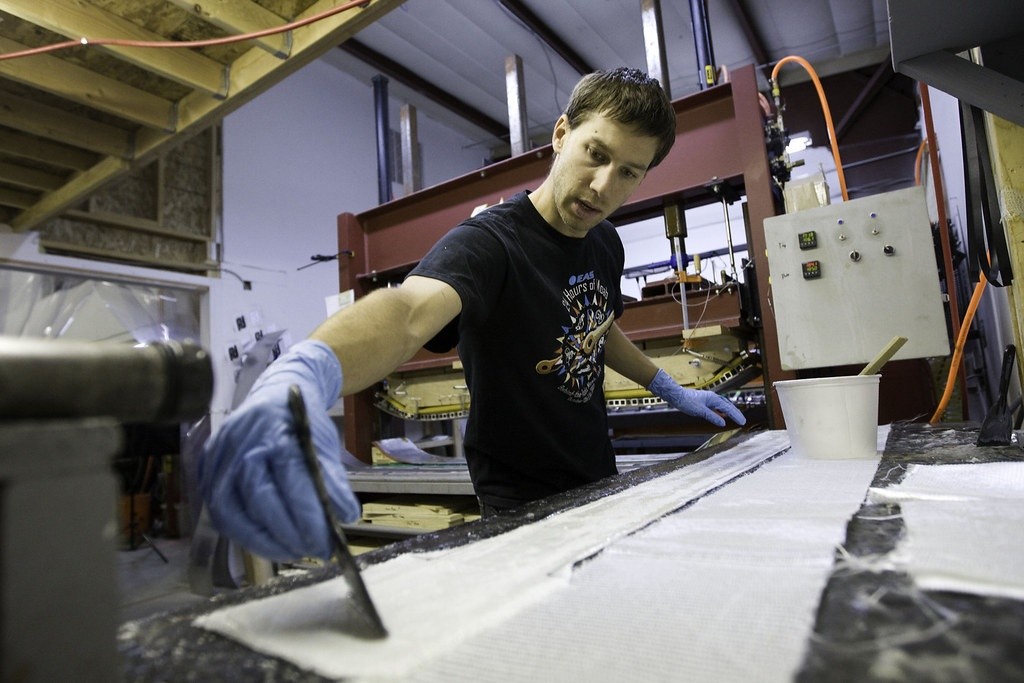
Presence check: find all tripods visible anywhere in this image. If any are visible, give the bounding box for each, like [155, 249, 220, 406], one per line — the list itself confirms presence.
[117, 494, 168, 563]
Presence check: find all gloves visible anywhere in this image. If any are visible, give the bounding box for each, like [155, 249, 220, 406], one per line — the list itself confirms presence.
[200, 339, 361, 564]
[646, 368, 746, 425]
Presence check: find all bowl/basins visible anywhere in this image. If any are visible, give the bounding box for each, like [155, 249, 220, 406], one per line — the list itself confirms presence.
[773, 374, 880, 460]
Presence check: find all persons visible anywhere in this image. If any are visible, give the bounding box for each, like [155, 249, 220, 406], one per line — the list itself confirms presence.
[198, 67, 749, 566]
